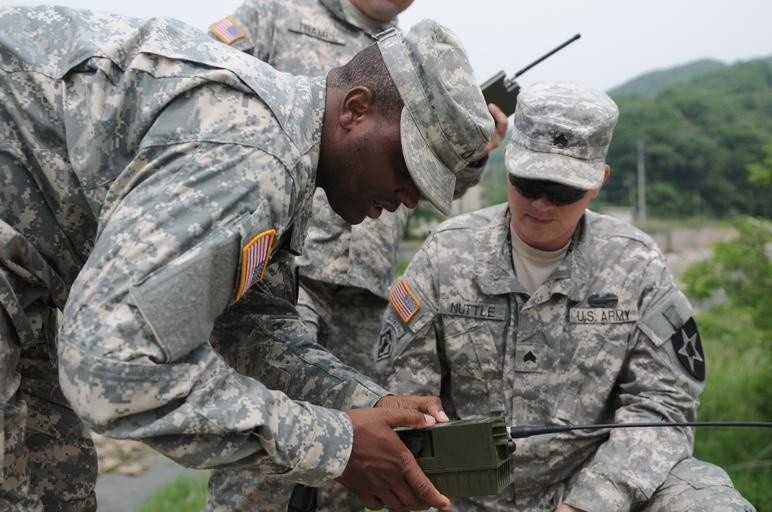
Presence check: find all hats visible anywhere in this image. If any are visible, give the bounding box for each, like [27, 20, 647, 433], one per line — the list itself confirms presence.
[505, 78, 619, 191]
[374, 17, 496, 216]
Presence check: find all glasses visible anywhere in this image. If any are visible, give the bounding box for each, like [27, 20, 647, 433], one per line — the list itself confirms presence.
[507, 173, 588, 206]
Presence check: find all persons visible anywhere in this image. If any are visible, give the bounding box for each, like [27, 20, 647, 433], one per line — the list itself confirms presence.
[204, 0, 509, 511]
[0, 2, 497, 512]
[373, 80, 760, 512]
